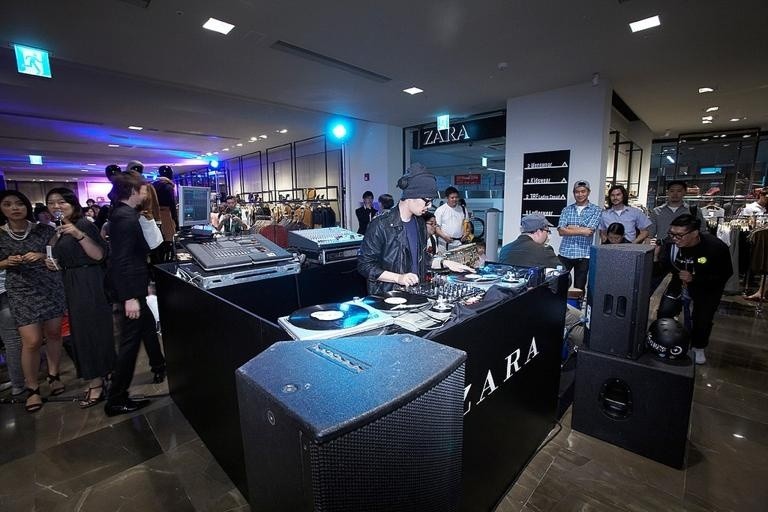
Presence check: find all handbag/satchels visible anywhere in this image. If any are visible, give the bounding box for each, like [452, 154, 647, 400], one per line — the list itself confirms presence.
[463, 219, 475, 242]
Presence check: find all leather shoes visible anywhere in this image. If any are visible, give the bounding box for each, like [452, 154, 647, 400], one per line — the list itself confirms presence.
[104, 399, 150, 417]
[154, 372, 163, 384]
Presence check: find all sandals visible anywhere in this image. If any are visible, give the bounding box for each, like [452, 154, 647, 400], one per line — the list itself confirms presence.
[78, 380, 106, 409]
[47, 372, 65, 396]
[25, 387, 42, 413]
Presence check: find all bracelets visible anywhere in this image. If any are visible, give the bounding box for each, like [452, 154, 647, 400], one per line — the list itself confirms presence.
[440, 259, 444, 269]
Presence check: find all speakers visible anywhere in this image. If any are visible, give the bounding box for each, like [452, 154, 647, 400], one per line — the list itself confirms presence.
[571, 349, 697, 471]
[234, 334, 468, 512]
[588, 243, 657, 361]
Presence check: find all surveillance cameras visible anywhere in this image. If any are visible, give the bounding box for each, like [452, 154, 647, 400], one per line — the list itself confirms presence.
[467, 141, 474, 146]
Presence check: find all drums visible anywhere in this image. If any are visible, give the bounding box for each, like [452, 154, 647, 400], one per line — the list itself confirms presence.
[562, 287, 583, 362]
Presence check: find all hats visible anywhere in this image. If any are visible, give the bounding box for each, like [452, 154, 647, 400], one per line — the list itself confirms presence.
[521, 213, 554, 232]
[399, 161, 437, 199]
[128, 160, 145, 170]
[573, 180, 591, 191]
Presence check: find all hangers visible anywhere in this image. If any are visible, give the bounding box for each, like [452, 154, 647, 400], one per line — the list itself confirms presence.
[700, 196, 768, 232]
[239, 202, 332, 230]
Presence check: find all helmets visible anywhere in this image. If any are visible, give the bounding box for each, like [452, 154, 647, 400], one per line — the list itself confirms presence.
[647, 317, 689, 361]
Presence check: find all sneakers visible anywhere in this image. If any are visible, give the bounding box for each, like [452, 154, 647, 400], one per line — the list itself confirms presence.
[692, 347, 706, 365]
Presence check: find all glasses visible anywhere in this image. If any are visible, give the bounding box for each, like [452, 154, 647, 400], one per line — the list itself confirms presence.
[667, 227, 692, 239]
[426, 222, 436, 226]
[542, 229, 551, 234]
[421, 197, 434, 207]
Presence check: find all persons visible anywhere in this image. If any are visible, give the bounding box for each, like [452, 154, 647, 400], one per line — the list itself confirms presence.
[216, 196, 248, 233]
[657, 213, 732, 364]
[433, 186, 470, 252]
[598, 184, 653, 244]
[354, 190, 378, 235]
[500, 213, 584, 346]
[420, 210, 448, 258]
[372, 193, 395, 220]
[742, 188, 767, 302]
[647, 179, 703, 290]
[557, 180, 600, 294]
[356, 161, 478, 296]
[472, 237, 486, 267]
[1, 161, 179, 418]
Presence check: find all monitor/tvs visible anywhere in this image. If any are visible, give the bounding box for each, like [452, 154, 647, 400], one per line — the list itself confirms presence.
[179, 186, 211, 227]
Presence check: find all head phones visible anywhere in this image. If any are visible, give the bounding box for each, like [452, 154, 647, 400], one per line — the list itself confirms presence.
[396, 172, 437, 190]
